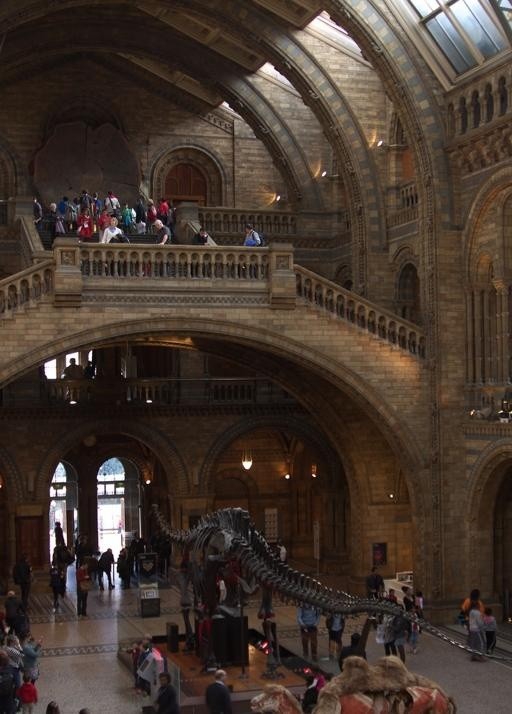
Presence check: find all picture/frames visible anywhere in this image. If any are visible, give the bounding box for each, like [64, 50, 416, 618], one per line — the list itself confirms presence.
[372, 542, 387, 567]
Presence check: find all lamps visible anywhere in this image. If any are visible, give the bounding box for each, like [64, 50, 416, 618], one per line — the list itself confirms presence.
[241, 447, 255, 472]
[321, 171, 334, 182]
[376, 141, 389, 154]
[275, 194, 287, 203]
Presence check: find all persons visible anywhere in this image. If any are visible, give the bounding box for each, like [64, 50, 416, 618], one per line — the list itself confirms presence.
[302, 676, 320, 713]
[50, 523, 113, 616]
[127, 633, 179, 713]
[63, 357, 96, 379]
[31, 191, 262, 277]
[2, 551, 42, 713]
[205, 670, 231, 714]
[367, 568, 424, 662]
[297, 602, 367, 670]
[118, 531, 170, 589]
[460, 589, 498, 661]
